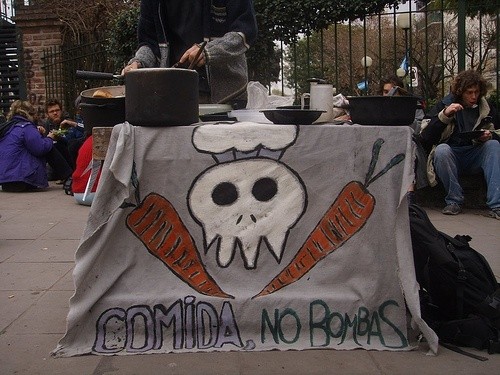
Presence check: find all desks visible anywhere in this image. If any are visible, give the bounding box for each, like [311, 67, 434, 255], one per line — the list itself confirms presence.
[50, 126, 440, 359]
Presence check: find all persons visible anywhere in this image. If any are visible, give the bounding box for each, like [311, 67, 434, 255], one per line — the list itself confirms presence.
[0, 99, 104, 206]
[335, 69, 500, 219]
[118, 0, 258, 111]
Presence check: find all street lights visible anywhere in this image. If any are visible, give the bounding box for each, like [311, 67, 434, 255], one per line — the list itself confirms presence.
[397, 13, 414, 92]
[361, 56, 373, 96]
[396, 68, 406, 81]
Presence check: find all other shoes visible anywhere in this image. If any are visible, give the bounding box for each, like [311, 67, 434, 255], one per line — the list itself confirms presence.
[441, 203, 463, 215]
[487, 207, 500, 220]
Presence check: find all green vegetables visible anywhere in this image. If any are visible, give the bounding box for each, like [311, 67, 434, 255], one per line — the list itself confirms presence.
[56, 129, 68, 136]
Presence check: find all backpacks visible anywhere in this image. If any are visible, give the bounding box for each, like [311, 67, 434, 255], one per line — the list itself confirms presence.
[406, 202, 500, 363]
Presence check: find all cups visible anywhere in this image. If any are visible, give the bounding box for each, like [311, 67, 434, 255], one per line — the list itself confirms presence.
[301, 78, 333, 125]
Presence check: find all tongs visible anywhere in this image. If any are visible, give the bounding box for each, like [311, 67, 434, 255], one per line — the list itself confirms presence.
[200, 110, 237, 122]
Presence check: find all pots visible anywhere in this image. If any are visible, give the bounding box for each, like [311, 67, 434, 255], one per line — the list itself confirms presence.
[74, 85, 125, 136]
[78, 68, 200, 127]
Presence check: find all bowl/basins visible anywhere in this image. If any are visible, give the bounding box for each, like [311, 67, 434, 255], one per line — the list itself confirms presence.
[456, 131, 485, 139]
[346, 94, 420, 126]
[259, 109, 327, 125]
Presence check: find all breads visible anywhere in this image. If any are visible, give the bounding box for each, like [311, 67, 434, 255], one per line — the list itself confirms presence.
[93, 89, 113, 98]
